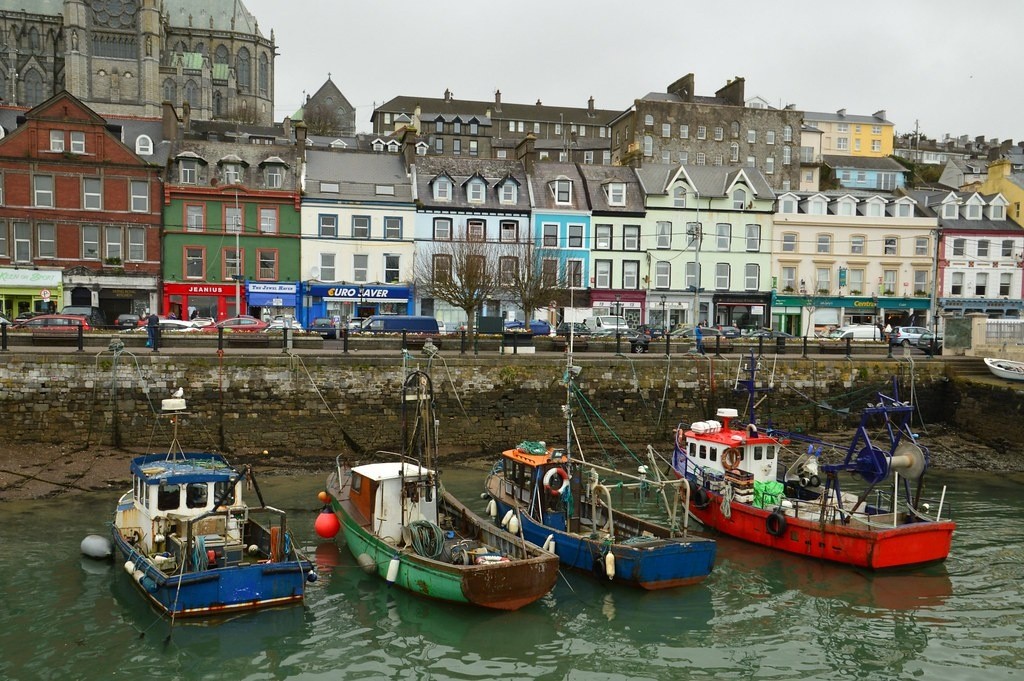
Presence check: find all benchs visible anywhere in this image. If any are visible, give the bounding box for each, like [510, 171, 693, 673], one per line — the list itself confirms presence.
[227, 333, 269, 347]
[402, 336, 442, 350]
[702, 342, 733, 353]
[551, 338, 589, 352]
[32, 328, 79, 346]
[818, 341, 851, 354]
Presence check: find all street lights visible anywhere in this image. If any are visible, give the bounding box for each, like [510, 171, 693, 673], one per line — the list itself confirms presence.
[932, 310, 940, 355]
[660, 294, 667, 338]
[358, 284, 366, 334]
[613, 294, 624, 338]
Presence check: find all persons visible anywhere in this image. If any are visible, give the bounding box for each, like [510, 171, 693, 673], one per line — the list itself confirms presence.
[147, 314, 159, 347]
[693, 319, 737, 354]
[874, 320, 892, 342]
[168, 312, 176, 319]
[191, 310, 200, 320]
[581, 319, 587, 329]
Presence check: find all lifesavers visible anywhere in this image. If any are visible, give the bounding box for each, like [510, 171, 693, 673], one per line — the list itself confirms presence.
[766, 513, 786, 536]
[809, 475, 821, 487]
[684, 481, 697, 500]
[678, 428, 683, 445]
[693, 490, 708, 510]
[543, 468, 570, 496]
[142, 467, 166, 474]
[722, 448, 741, 469]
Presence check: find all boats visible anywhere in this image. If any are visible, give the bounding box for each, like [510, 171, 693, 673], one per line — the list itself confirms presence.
[483, 341, 716, 591]
[672, 346, 954, 569]
[324, 372, 559, 612]
[109, 397, 319, 620]
[984, 356, 1024, 381]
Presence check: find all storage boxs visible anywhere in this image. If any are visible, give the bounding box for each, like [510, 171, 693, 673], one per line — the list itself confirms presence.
[147, 552, 174, 570]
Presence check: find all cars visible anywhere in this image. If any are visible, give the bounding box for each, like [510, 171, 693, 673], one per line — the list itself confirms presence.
[1, 306, 942, 347]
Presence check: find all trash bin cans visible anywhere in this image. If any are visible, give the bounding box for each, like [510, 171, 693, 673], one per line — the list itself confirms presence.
[776, 337, 786, 354]
